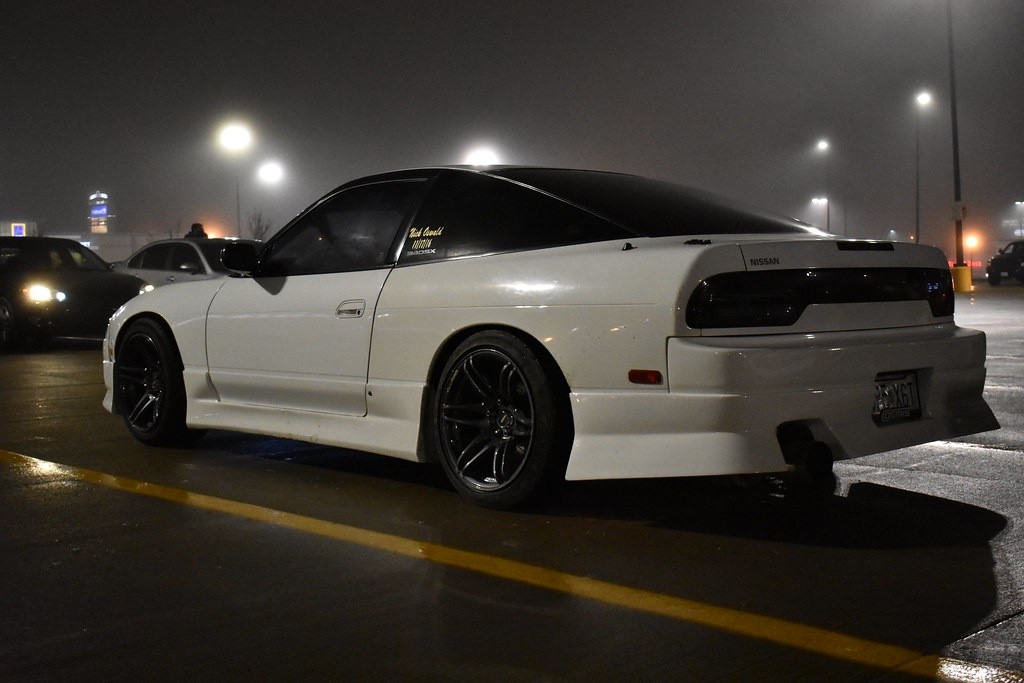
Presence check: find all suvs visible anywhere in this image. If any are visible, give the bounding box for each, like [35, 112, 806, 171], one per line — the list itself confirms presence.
[986, 240, 1024, 286]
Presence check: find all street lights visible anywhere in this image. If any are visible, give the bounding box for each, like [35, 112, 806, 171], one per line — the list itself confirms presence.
[819, 134, 830, 231]
[914, 90, 930, 242]
[966, 235, 976, 278]
[221, 125, 249, 240]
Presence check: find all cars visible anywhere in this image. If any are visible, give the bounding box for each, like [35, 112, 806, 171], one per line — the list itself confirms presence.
[0, 236, 154, 352]
[114, 238, 264, 288]
[102, 164, 999, 520]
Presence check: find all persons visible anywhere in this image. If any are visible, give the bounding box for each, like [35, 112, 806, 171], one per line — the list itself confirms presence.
[182, 223, 206, 238]
